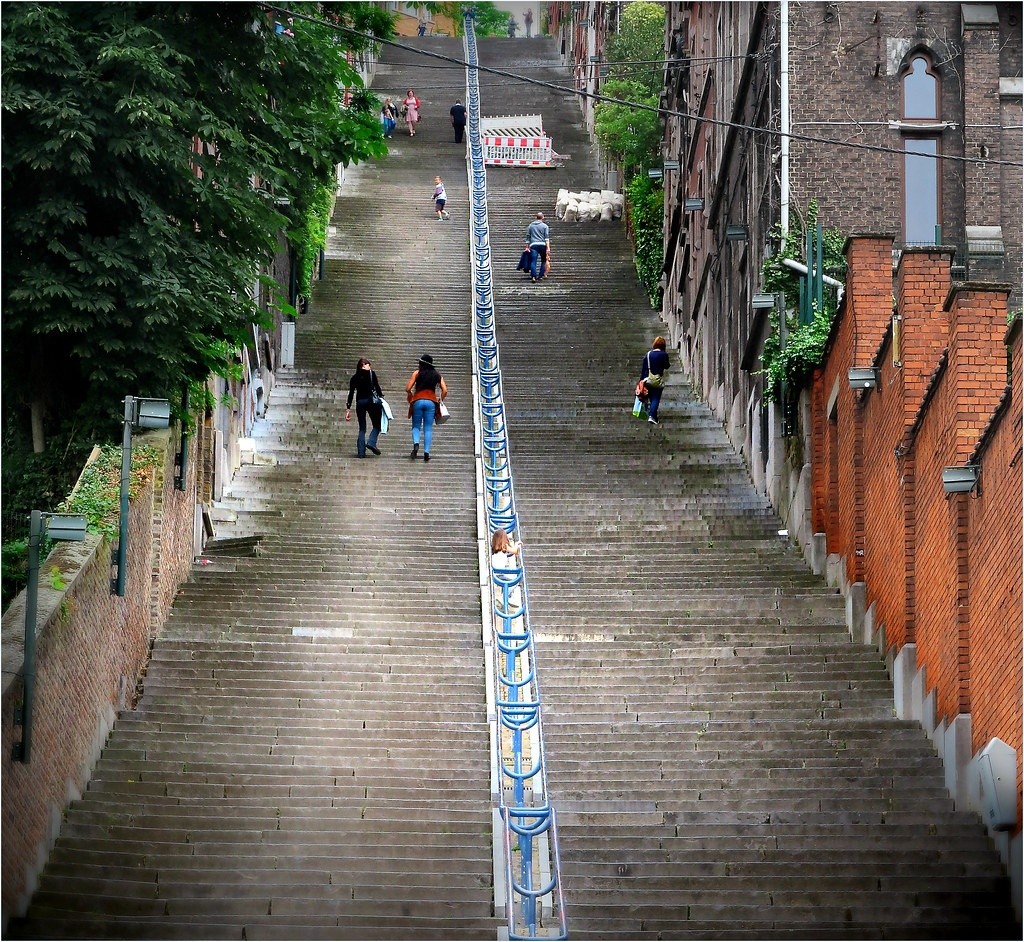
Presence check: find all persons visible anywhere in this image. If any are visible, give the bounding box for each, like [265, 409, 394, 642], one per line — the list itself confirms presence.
[492, 529, 524, 569]
[463, 3, 476, 19]
[508, 14, 521, 38]
[525, 212, 550, 282]
[639, 336, 671, 424]
[431, 175, 450, 222]
[416, 12, 432, 37]
[403, 89, 420, 137]
[450, 98, 467, 143]
[380, 96, 399, 140]
[345, 359, 385, 459]
[522, 8, 533, 38]
[405, 354, 447, 462]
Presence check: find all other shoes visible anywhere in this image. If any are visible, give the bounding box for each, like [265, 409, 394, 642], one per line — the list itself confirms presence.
[436, 216, 443, 222]
[648, 414, 661, 426]
[531, 275, 544, 282]
[408, 131, 417, 136]
[364, 441, 381, 455]
[446, 211, 451, 220]
[356, 452, 368, 459]
[410, 444, 420, 459]
[422, 452, 431, 462]
[643, 402, 649, 410]
[384, 133, 394, 138]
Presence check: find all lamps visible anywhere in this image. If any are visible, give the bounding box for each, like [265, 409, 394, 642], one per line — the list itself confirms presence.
[590, 55, 600, 63]
[578, 19, 589, 27]
[573, 3, 582, 9]
[648, 168, 663, 177]
[845, 366, 880, 391]
[599, 67, 610, 75]
[725, 224, 751, 241]
[941, 463, 983, 499]
[663, 160, 680, 170]
[686, 198, 705, 212]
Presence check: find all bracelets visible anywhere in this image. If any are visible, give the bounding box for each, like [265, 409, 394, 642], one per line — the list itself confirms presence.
[526, 247, 529, 248]
[545, 250, 550, 252]
[409, 391, 412, 396]
[345, 409, 351, 411]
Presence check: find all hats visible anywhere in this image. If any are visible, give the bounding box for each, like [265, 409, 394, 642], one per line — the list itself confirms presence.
[414, 353, 439, 368]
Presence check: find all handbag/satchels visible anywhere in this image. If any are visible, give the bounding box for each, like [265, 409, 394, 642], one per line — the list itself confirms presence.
[646, 371, 668, 389]
[370, 388, 383, 409]
[436, 393, 451, 426]
